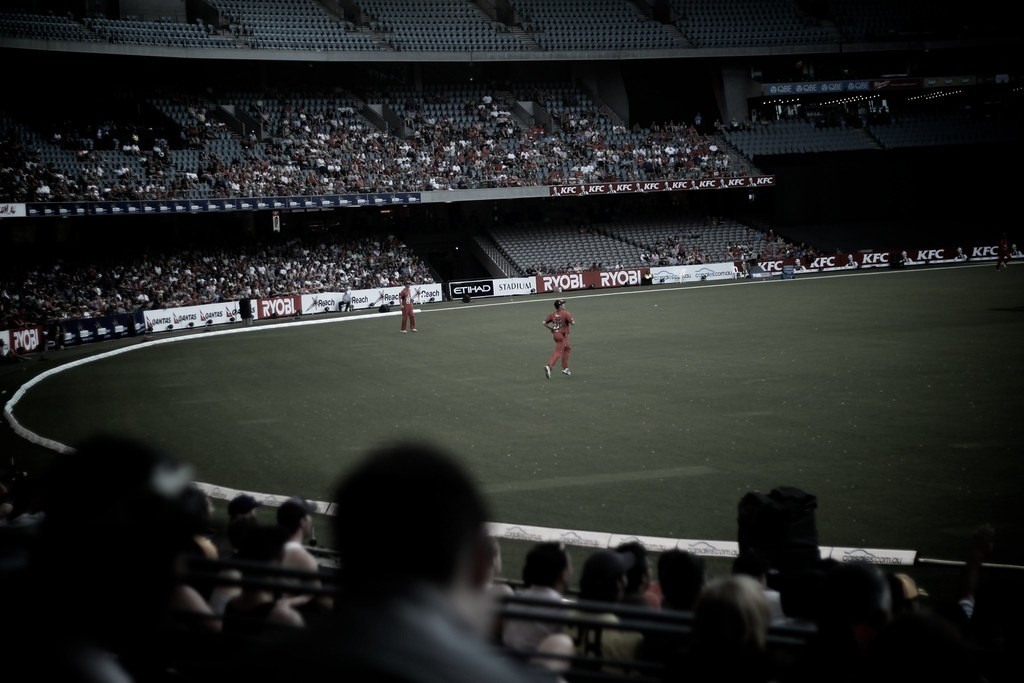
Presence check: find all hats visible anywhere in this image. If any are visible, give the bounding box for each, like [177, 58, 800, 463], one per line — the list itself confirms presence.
[276, 496, 318, 518]
[405, 280, 412, 285]
[227, 494, 263, 516]
[554, 299, 567, 306]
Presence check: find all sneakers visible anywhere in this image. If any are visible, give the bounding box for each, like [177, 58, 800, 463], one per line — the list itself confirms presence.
[410, 328, 418, 332]
[562, 367, 571, 375]
[545, 365, 551, 379]
[400, 329, 407, 332]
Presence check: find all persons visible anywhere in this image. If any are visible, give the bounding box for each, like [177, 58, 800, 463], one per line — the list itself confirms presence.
[994, 229, 1013, 270]
[0, 430, 1021, 683]
[543, 298, 575, 378]
[0, 91, 904, 201]
[1, 338, 33, 364]
[47, 318, 66, 351]
[399, 281, 420, 334]
[0, 214, 847, 328]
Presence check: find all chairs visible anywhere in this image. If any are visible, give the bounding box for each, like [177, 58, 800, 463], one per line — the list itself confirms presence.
[0, 0, 1023, 683]
